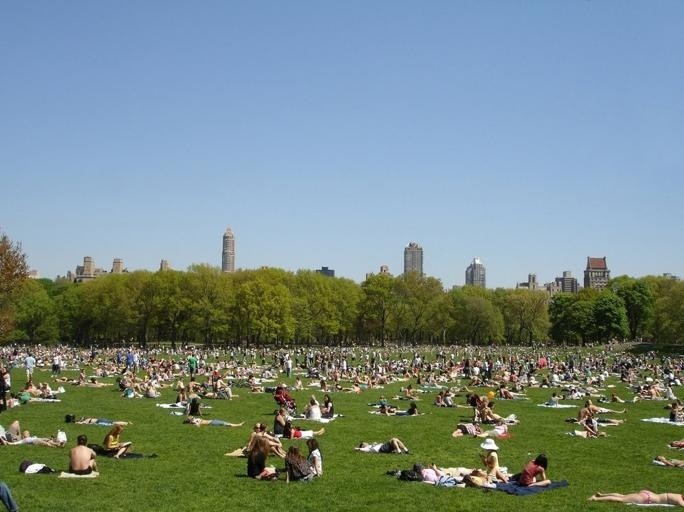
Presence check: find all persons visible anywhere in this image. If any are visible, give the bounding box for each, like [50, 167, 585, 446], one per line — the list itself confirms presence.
[1, 336, 334, 419]
[186, 416, 244, 426]
[0, 417, 130, 475]
[588, 441, 683, 504]
[583, 417, 598, 437]
[358, 422, 550, 487]
[332, 341, 683, 424]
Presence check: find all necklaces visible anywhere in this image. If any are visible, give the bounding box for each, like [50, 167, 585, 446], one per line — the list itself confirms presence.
[245, 409, 323, 480]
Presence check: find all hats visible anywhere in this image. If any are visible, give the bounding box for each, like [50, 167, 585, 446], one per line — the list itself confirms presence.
[480, 438, 499, 450]
